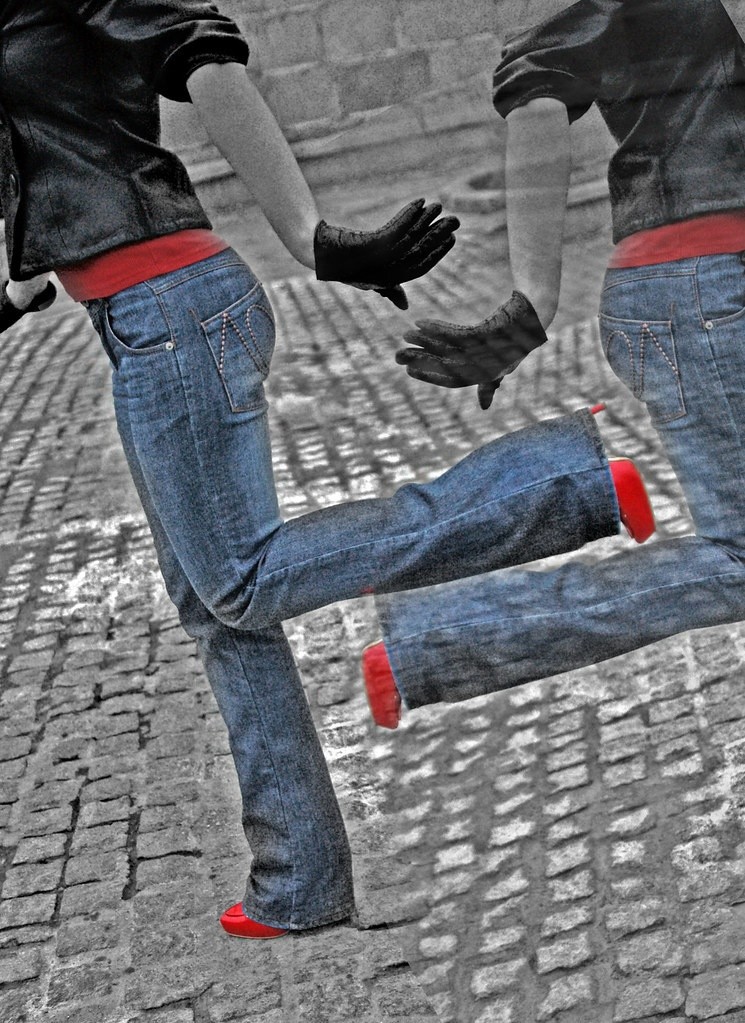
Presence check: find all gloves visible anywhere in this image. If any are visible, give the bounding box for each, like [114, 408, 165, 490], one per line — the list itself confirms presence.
[0, 277, 58, 335]
[313, 197, 462, 310]
[394, 290, 547, 411]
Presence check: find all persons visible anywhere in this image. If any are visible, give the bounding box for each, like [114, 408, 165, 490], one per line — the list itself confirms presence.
[363, 0, 745, 729]
[0, 0, 656, 939]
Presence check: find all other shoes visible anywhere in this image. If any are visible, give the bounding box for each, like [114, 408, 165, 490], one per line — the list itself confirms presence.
[610, 456, 655, 543]
[220, 900, 288, 938]
[364, 639, 403, 730]
